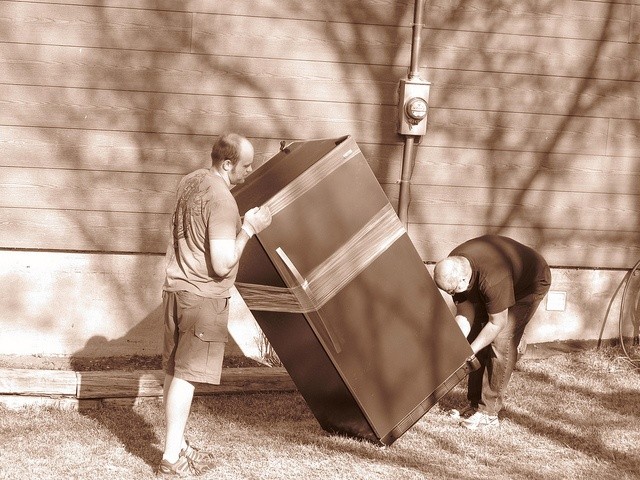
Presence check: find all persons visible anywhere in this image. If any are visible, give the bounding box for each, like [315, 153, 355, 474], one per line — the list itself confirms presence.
[155, 131, 272, 476]
[434, 236, 550, 430]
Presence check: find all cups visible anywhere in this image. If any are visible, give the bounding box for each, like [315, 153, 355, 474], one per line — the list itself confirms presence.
[447, 279, 465, 296]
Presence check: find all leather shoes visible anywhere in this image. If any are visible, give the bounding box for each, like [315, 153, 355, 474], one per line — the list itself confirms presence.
[241, 206, 273, 239]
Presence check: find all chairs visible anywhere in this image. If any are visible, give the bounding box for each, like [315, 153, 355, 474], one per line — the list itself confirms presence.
[460, 411, 499, 430]
[178, 439, 217, 465]
[449, 400, 478, 419]
[157, 449, 209, 479]
